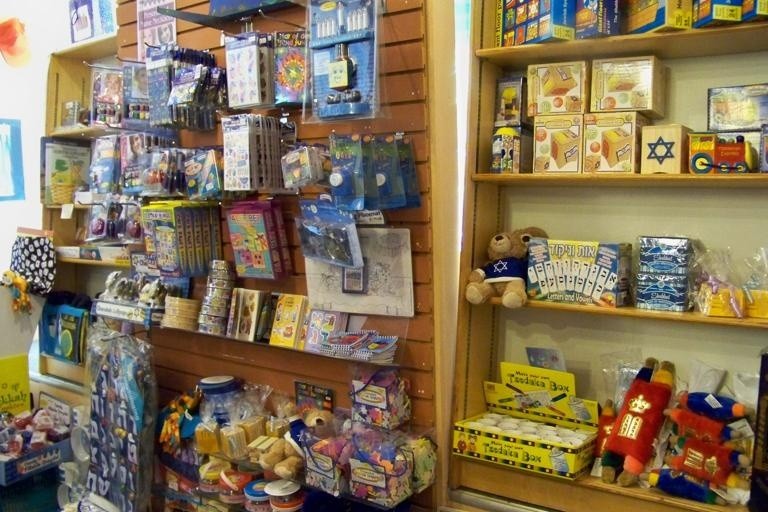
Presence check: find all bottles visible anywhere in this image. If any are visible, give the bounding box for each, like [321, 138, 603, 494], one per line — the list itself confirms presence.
[198, 376, 244, 429]
[197, 460, 308, 512]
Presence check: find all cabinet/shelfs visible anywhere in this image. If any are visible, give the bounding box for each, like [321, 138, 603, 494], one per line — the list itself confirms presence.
[448, 0, 768, 512]
[32, 32, 133, 511]
